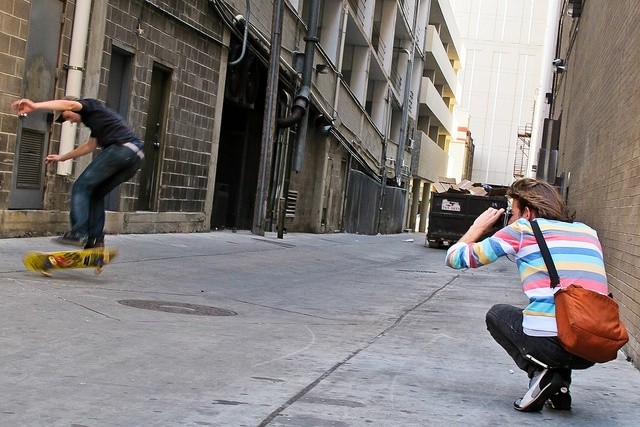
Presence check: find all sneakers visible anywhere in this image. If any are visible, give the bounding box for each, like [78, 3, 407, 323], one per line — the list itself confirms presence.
[50, 235, 87, 247]
[514, 368, 562, 412]
[545, 385, 571, 410]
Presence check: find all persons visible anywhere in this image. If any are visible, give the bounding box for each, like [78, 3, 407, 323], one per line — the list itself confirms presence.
[447, 178, 611, 415]
[9, 94, 146, 252]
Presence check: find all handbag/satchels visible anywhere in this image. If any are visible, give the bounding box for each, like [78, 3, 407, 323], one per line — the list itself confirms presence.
[530, 219, 629, 363]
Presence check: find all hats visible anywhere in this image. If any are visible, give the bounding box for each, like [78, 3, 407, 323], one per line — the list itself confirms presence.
[55, 95, 78, 124]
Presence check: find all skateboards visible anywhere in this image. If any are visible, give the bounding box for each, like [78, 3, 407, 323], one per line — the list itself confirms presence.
[22, 248, 118, 277]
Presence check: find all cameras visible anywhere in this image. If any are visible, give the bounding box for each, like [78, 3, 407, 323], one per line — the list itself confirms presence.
[492, 213, 515, 228]
